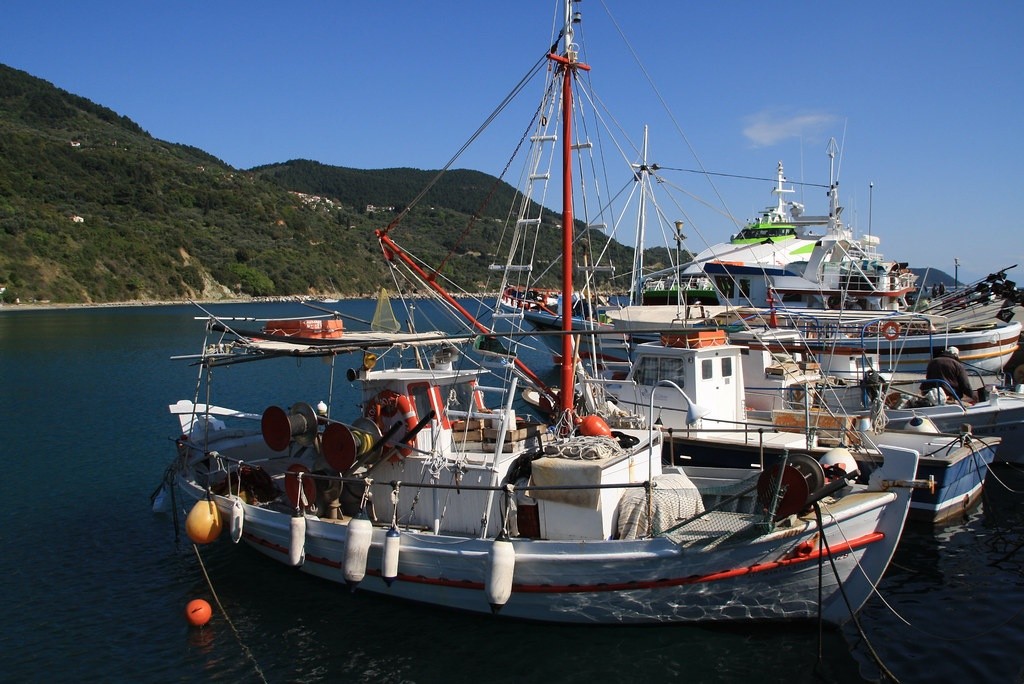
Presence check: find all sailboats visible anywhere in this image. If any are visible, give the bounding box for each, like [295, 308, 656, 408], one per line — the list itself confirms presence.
[683, 162, 918, 309]
[169, 0, 920, 627]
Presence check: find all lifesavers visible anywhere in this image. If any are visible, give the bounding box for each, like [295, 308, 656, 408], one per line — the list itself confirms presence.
[365, 389, 419, 464]
[882, 321, 901, 340]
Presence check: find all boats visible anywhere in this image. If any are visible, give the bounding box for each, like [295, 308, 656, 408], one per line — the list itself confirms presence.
[897, 259, 917, 305]
[191, 296, 489, 356]
[522, 238, 1002, 524]
[523, 302, 1023, 372]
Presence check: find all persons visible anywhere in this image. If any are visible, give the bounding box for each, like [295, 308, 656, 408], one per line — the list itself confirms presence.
[932, 281, 945, 298]
[919, 345, 978, 405]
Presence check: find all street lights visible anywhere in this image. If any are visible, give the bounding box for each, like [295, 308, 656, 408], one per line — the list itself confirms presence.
[953, 256, 960, 289]
[673, 215, 687, 248]
